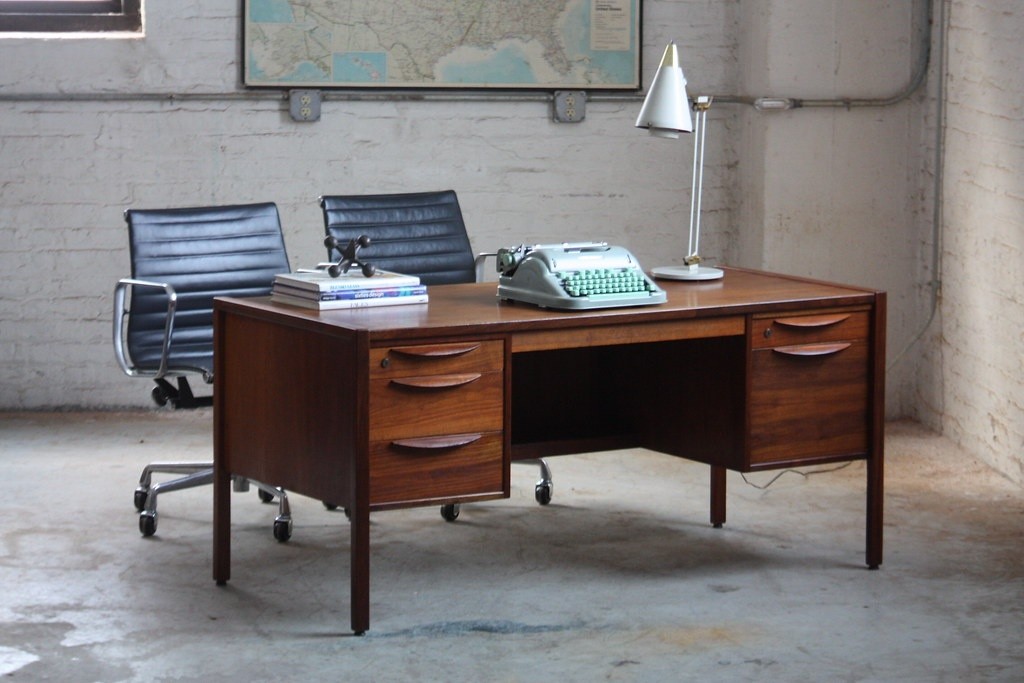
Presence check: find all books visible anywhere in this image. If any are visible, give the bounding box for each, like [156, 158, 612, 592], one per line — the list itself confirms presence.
[271, 262, 430, 311]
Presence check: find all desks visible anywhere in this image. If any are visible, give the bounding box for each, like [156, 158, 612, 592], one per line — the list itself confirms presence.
[211, 265, 888, 636]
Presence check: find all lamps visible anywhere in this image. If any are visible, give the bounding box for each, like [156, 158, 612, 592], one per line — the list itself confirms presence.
[635, 39, 724, 282]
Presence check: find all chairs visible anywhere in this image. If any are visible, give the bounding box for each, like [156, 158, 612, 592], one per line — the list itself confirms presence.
[317, 189, 553, 523]
[112, 201, 337, 542]
